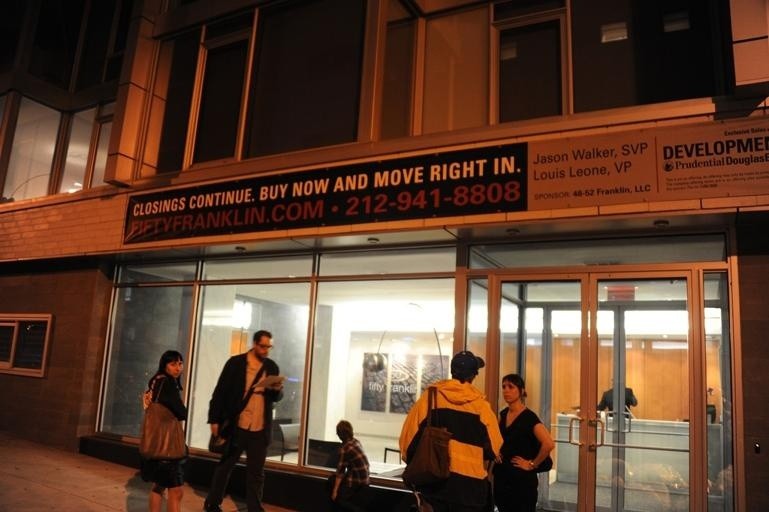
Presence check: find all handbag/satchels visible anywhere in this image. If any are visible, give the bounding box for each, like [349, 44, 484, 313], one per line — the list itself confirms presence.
[208, 417, 235, 454]
[139, 379, 186, 461]
[406, 386, 453, 486]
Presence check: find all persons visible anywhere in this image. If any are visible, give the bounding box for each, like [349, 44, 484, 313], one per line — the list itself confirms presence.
[202, 328, 283, 512]
[143, 350, 189, 511]
[396, 349, 503, 512]
[486, 375, 557, 512]
[598, 377, 638, 418]
[325, 419, 371, 512]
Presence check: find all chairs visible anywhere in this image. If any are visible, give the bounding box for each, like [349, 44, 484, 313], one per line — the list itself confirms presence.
[308, 439, 342, 469]
[280, 423, 302, 462]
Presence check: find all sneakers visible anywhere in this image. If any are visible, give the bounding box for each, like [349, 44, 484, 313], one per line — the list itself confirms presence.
[204, 499, 223, 512]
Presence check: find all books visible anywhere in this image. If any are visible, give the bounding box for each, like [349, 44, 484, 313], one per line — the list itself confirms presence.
[252, 375, 286, 390]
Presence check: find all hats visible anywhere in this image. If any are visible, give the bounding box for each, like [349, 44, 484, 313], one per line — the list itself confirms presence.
[450, 350, 486, 379]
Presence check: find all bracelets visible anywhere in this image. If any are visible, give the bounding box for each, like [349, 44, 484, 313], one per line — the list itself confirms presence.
[528, 459, 536, 470]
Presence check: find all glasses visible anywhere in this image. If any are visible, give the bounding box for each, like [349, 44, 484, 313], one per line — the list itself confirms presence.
[255, 343, 273, 349]
